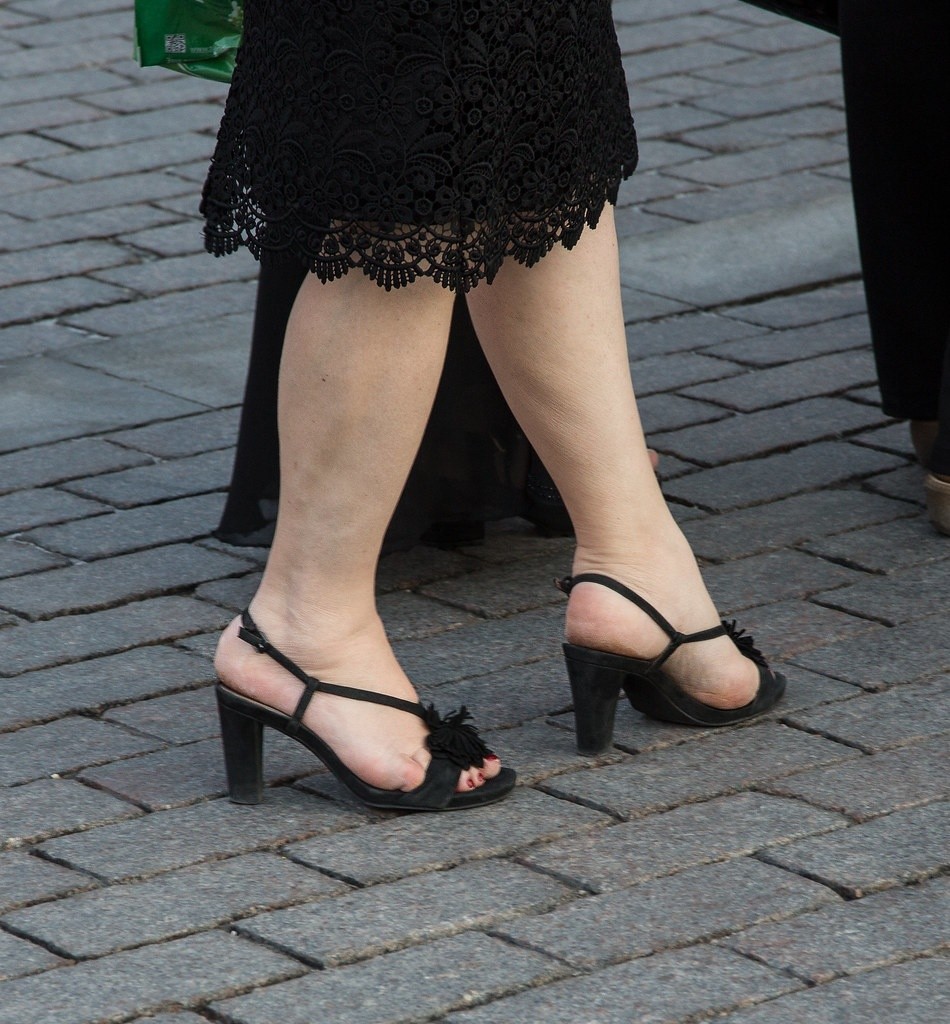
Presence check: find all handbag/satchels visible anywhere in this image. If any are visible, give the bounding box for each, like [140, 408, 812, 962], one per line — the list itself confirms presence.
[134, 0, 242, 80]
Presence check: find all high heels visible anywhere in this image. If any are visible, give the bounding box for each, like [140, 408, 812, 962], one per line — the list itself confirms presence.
[216, 605, 516, 813]
[558, 571, 785, 756]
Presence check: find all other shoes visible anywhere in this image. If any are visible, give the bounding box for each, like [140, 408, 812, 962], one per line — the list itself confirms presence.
[521, 454, 574, 536]
[923, 474, 950, 530]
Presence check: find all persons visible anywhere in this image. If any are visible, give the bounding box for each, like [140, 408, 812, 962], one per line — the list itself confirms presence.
[198, 1, 791, 811]
[748, 0, 950, 548]
